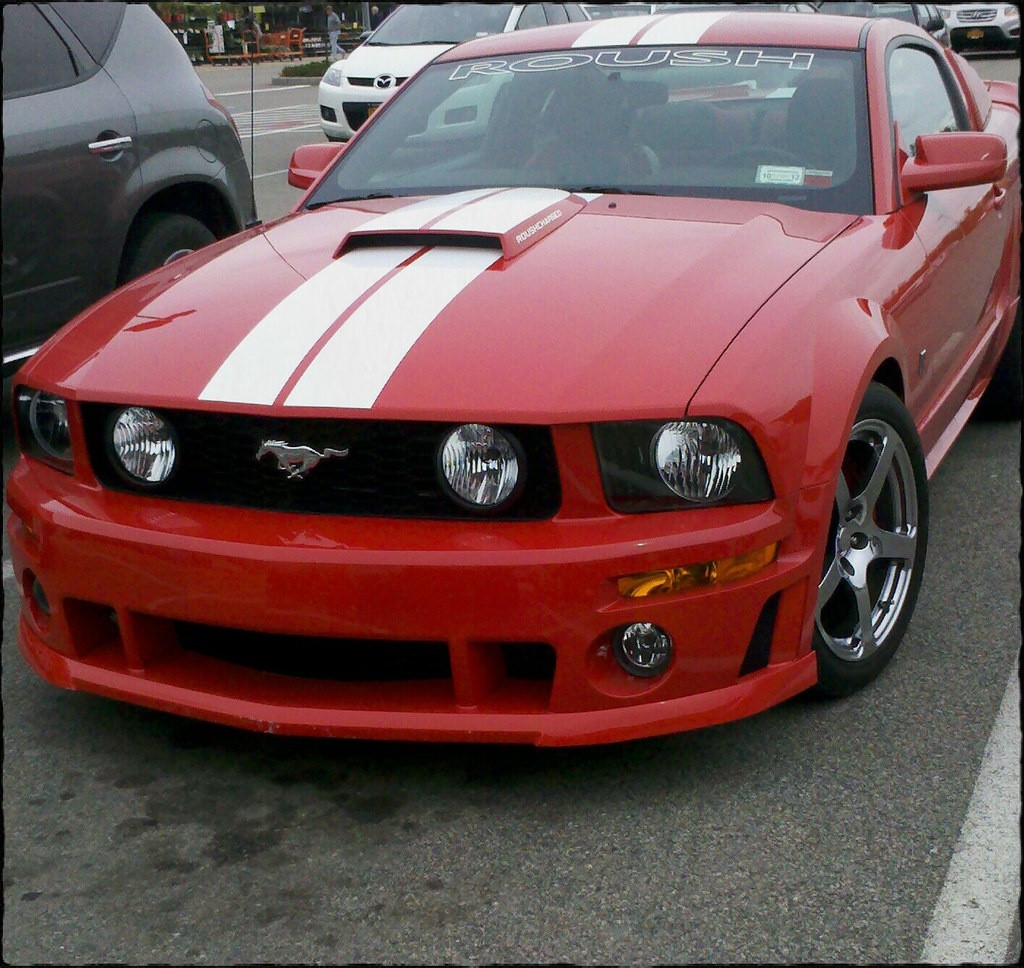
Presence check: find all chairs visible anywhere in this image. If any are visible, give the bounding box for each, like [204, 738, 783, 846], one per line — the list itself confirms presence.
[781, 77, 859, 174]
[525, 77, 664, 185]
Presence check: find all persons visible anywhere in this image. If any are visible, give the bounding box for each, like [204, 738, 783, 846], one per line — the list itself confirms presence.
[370, 6, 380, 31]
[326, 6, 346, 59]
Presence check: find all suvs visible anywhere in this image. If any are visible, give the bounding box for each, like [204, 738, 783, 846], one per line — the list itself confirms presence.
[317, 3, 597, 144]
[0, 0, 262, 383]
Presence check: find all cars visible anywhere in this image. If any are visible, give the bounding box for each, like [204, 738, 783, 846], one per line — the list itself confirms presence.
[581, 1, 1021, 56]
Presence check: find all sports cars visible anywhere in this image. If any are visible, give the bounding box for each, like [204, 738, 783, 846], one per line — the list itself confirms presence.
[5, 7, 1021, 747]
